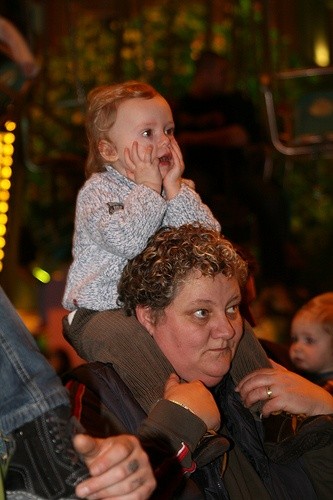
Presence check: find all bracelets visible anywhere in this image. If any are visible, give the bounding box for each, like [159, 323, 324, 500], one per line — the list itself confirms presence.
[169, 400, 196, 414]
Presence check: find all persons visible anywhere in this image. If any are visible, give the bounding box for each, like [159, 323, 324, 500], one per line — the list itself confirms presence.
[61, 79, 326, 469]
[175, 50, 290, 269]
[0, 288, 156, 500]
[290, 292, 333, 394]
[59, 224, 333, 500]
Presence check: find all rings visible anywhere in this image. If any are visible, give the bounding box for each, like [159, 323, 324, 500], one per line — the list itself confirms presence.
[267, 387, 272, 398]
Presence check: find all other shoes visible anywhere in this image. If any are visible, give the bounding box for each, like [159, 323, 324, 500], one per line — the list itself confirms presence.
[6, 406, 92, 500]
[263, 412, 333, 465]
[191, 430, 230, 469]
